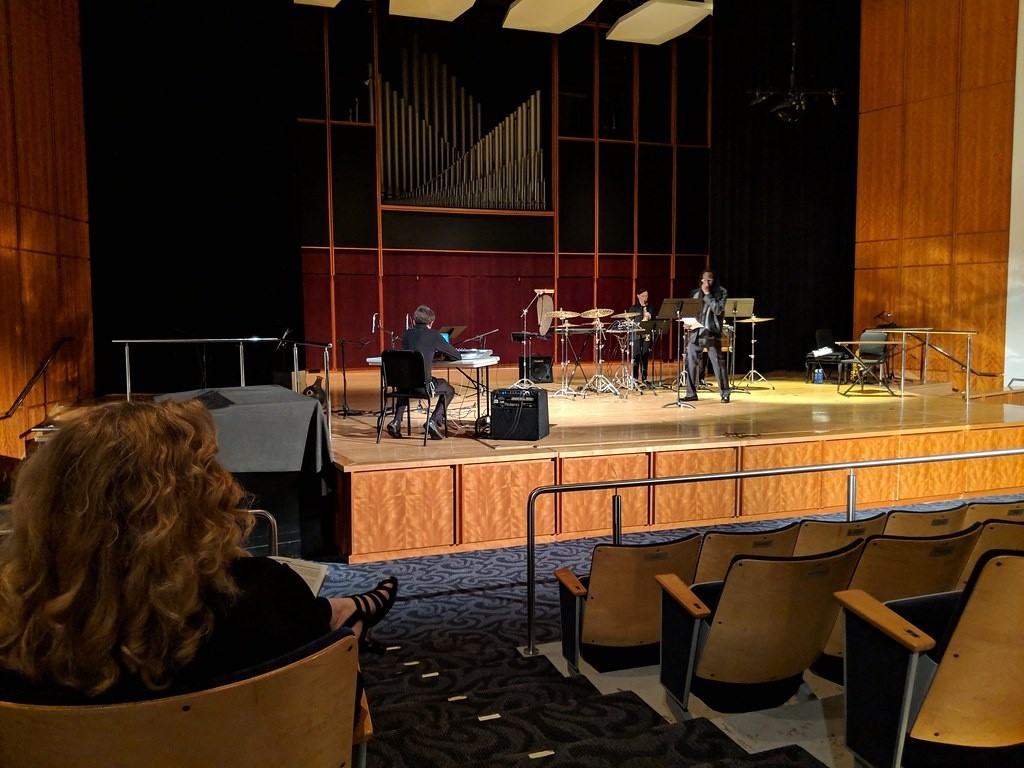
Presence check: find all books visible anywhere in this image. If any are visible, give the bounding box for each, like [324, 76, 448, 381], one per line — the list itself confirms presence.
[267, 556, 328, 597]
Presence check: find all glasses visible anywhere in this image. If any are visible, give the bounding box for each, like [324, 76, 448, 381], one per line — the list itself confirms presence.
[701, 278, 714, 283]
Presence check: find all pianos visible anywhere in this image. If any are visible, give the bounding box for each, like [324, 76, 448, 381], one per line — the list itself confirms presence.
[554, 328, 607, 390]
[364, 347, 500, 436]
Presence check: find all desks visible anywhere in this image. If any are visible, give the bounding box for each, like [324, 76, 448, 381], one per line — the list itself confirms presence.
[366, 356, 501, 438]
[835, 327, 979, 405]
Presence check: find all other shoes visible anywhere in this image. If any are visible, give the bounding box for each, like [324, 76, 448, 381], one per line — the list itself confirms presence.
[680, 393, 698, 401]
[721, 393, 729, 403]
[643, 381, 655, 389]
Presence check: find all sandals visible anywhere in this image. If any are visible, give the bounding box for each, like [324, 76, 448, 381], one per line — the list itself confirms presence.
[336, 575, 399, 646]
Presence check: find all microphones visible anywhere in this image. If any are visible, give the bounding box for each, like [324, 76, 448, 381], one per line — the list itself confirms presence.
[874, 311, 886, 319]
[539, 290, 546, 298]
[372, 315, 376, 334]
[406, 314, 409, 331]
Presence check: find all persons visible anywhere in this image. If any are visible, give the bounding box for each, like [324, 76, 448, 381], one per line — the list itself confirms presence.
[0, 402, 398, 707]
[629, 288, 656, 382]
[679, 270, 730, 403]
[387, 305, 462, 439]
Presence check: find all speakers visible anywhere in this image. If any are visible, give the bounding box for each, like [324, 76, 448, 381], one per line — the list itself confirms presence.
[490, 388, 550, 441]
[518, 354, 555, 384]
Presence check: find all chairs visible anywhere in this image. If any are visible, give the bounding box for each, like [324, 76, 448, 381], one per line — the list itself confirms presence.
[552, 496, 1024, 767]
[803, 329, 889, 391]
[376, 350, 448, 447]
[0, 627, 373, 768]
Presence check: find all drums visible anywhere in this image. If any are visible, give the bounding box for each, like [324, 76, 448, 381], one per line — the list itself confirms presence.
[718, 324, 733, 353]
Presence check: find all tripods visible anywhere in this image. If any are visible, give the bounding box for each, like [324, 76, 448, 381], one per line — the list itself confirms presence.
[460, 329, 499, 399]
[881, 317, 914, 386]
[329, 338, 374, 418]
[551, 297, 703, 409]
[724, 298, 775, 391]
[385, 399, 434, 417]
[510, 293, 539, 389]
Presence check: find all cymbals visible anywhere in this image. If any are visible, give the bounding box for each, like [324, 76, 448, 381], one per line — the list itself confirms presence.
[545, 308, 641, 318]
[732, 318, 775, 323]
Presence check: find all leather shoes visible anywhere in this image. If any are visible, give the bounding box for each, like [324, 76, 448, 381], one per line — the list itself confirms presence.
[387, 420, 403, 439]
[423, 421, 444, 439]
